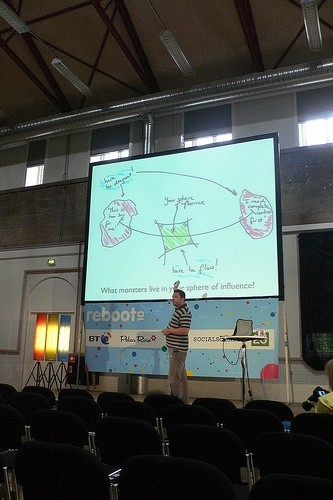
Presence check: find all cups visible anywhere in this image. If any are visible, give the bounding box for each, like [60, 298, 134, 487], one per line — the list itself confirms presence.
[258, 330, 265, 336]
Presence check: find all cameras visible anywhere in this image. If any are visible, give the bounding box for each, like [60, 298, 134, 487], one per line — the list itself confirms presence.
[302, 386, 329, 411]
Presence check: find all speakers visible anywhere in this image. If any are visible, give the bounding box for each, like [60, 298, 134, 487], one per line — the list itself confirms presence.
[67, 354, 98, 385]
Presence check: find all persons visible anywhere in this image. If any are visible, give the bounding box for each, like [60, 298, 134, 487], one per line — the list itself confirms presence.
[160, 280, 192, 404]
[307, 359, 333, 414]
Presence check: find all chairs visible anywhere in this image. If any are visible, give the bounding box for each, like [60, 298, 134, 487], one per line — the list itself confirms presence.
[0, 383, 333, 500]
